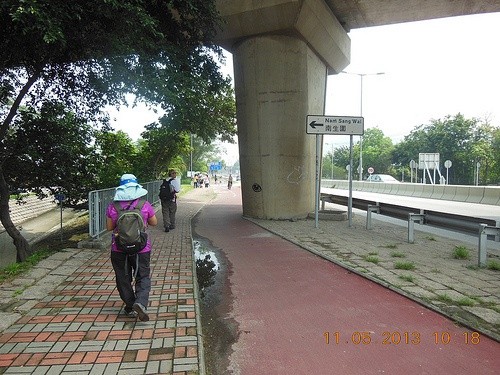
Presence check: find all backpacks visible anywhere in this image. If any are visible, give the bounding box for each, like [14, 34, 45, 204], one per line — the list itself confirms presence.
[158, 179, 175, 203]
[111, 199, 147, 255]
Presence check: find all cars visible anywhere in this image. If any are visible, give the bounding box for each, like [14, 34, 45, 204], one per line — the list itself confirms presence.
[365, 173, 400, 183]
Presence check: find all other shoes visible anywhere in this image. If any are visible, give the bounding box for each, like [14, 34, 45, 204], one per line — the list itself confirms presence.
[170, 225, 175, 229]
[133, 303, 150, 322]
[124, 310, 138, 318]
[165, 227, 169, 232]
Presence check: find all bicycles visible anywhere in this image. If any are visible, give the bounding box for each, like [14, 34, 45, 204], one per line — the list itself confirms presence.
[229, 183, 232, 189]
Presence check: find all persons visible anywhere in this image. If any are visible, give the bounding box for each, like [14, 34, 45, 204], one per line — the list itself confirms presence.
[193, 173, 209, 188]
[228, 174, 232, 190]
[159, 169, 180, 232]
[105, 174, 158, 322]
[215, 175, 223, 184]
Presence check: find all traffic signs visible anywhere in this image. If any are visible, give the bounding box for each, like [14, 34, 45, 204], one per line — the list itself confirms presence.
[305, 115, 364, 135]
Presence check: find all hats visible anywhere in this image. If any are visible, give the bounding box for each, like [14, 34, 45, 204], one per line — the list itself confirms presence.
[168, 169, 177, 174]
[115, 174, 144, 190]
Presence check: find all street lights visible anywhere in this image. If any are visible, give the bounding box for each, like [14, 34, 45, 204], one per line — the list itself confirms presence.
[324, 142, 334, 179]
[339, 70, 386, 182]
